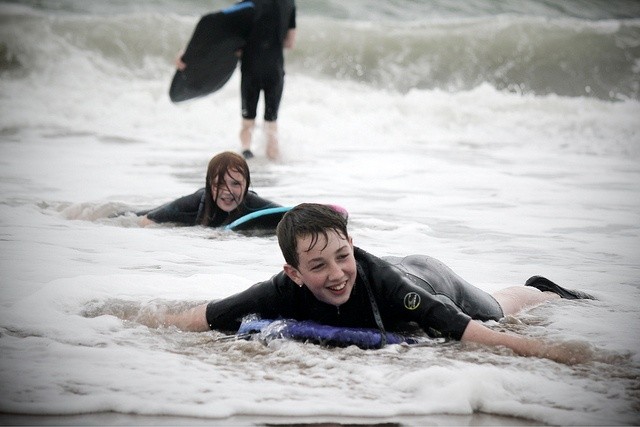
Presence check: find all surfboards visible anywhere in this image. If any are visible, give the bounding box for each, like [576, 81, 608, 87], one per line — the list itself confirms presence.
[225, 205, 347, 231]
[169, 2, 255, 103]
[237, 315, 429, 348]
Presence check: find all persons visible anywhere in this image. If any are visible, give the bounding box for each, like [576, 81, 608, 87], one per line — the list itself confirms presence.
[124, 203, 595, 366]
[100, 151, 291, 233]
[174, 1, 297, 164]
